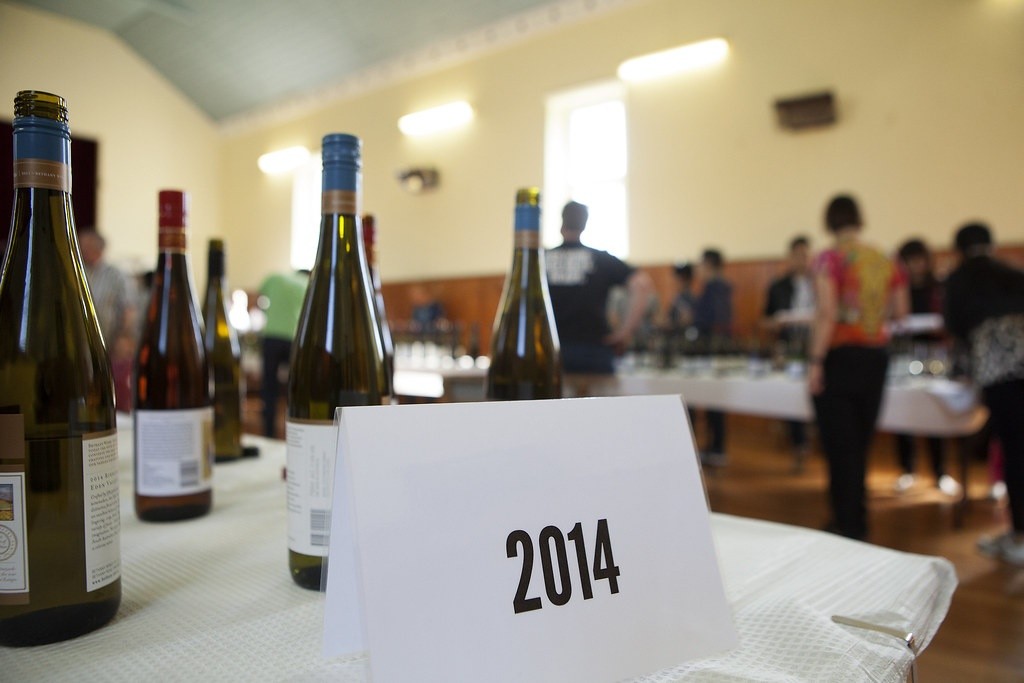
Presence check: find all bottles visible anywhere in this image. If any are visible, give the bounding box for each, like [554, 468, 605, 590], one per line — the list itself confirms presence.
[362, 214, 395, 387]
[284, 131, 393, 592]
[0, 89, 123, 649]
[201, 239, 244, 463]
[486, 187, 564, 403]
[131, 189, 216, 523]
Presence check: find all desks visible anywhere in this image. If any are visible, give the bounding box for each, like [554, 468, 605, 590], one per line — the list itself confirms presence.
[0, 345, 988, 683]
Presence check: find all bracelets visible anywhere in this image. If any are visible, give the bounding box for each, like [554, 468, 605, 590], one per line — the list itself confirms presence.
[809, 354, 825, 365]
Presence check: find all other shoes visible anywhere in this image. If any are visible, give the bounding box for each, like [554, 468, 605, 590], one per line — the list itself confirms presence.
[974, 533, 1024, 567]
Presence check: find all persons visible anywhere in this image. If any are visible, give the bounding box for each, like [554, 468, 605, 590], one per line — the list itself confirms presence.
[247, 269, 311, 439]
[82, 232, 137, 352]
[667, 249, 733, 463]
[891, 239, 949, 485]
[766, 236, 814, 451]
[408, 283, 447, 342]
[940, 224, 1024, 563]
[543, 200, 660, 397]
[807, 192, 906, 539]
